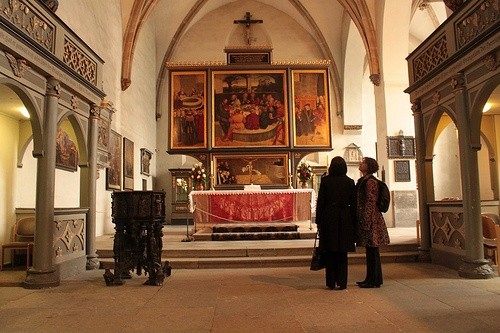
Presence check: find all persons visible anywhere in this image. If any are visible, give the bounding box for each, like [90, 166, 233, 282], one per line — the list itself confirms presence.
[355, 157, 390, 288]
[316, 156, 356, 290]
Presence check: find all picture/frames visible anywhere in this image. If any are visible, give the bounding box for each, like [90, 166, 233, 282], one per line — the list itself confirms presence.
[169, 69, 209, 150]
[290, 68, 333, 151]
[211, 67, 291, 150]
[55, 128, 78, 172]
[106, 129, 123, 190]
[210, 151, 292, 189]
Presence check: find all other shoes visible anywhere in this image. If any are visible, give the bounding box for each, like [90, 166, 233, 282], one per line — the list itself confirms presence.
[356, 280, 365, 284]
[360, 281, 380, 288]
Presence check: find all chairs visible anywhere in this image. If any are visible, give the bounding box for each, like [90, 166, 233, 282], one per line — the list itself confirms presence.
[482, 212, 500, 266]
[1, 216, 35, 271]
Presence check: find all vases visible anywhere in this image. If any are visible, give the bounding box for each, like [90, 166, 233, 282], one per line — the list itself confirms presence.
[196, 180, 204, 190]
[301, 180, 308, 189]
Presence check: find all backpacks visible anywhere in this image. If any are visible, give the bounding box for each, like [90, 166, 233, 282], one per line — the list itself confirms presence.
[362, 176, 390, 213]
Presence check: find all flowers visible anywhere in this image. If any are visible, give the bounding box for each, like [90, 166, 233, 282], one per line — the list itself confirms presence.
[188, 162, 207, 184]
[296, 162, 315, 182]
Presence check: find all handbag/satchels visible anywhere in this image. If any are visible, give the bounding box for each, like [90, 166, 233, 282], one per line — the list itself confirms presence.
[310, 233, 325, 270]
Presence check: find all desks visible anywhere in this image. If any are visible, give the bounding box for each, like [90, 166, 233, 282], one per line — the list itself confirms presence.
[186, 189, 316, 235]
